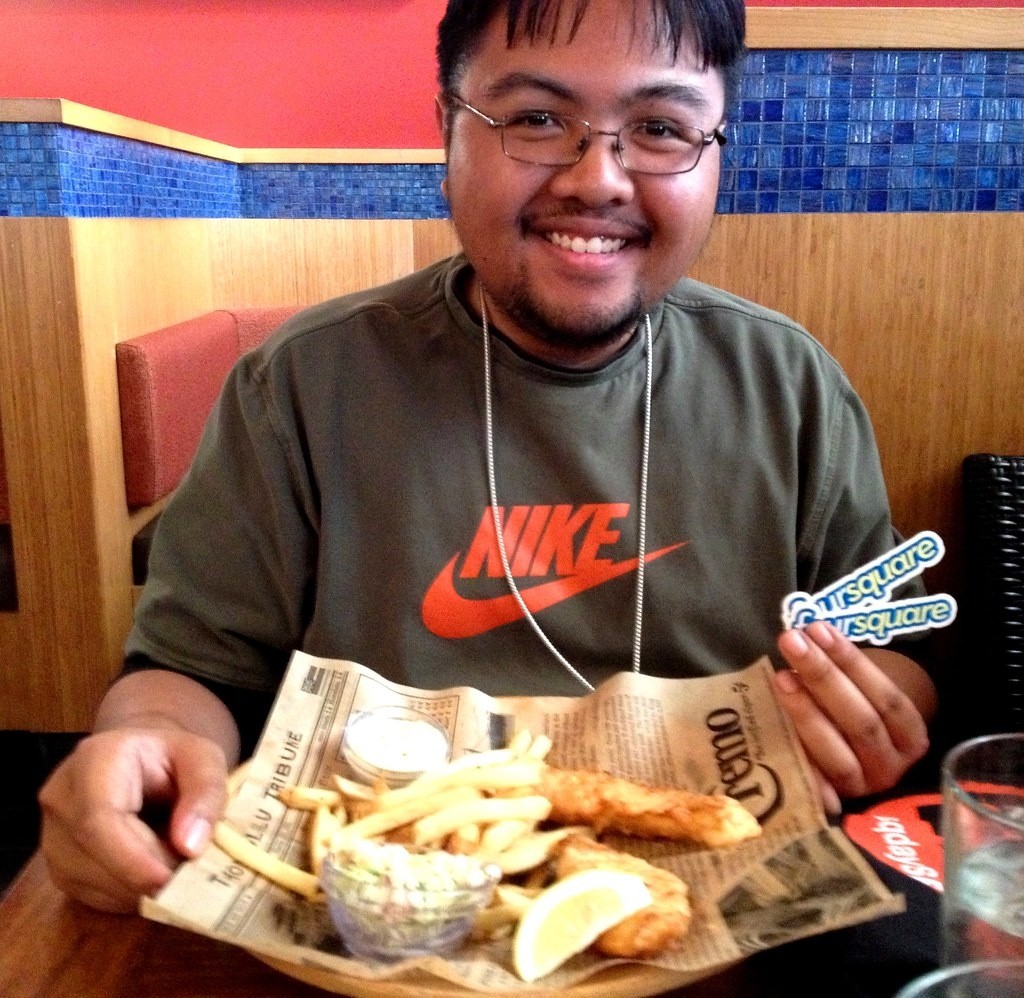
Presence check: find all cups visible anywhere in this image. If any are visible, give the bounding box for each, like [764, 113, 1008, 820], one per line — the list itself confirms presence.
[939, 732, 1024, 964]
[896, 959, 1024, 998]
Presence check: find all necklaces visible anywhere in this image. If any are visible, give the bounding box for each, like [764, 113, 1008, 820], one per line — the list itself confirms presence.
[480, 286, 651, 693]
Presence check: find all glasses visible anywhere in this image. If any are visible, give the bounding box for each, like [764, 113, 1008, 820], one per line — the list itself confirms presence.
[445, 88, 727, 174]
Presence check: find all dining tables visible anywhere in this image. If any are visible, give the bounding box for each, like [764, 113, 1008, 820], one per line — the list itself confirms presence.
[3, 765, 1024, 997]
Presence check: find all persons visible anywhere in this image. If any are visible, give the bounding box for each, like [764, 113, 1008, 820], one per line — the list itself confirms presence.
[40, 1, 935, 917]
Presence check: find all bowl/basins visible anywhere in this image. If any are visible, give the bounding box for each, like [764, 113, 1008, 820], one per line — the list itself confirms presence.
[321, 844, 501, 962]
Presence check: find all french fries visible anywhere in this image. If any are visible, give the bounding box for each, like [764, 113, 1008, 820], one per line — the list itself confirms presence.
[210, 732, 594, 945]
